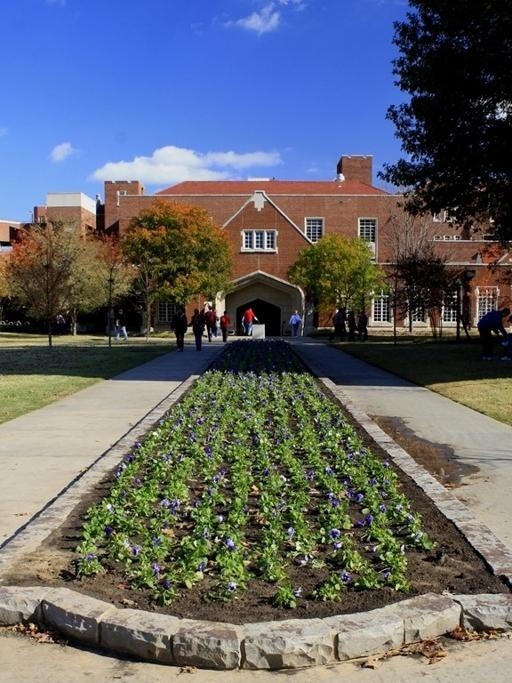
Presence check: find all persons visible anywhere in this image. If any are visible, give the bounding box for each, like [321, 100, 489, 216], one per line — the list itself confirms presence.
[170, 306, 187, 352]
[220, 311, 231, 342]
[477, 307, 510, 360]
[460, 308, 470, 332]
[189, 306, 219, 352]
[289, 310, 302, 338]
[114, 319, 121, 344]
[241, 306, 259, 336]
[329, 307, 368, 342]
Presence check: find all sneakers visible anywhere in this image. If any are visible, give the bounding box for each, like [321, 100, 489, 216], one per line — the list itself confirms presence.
[481, 356, 512, 361]
